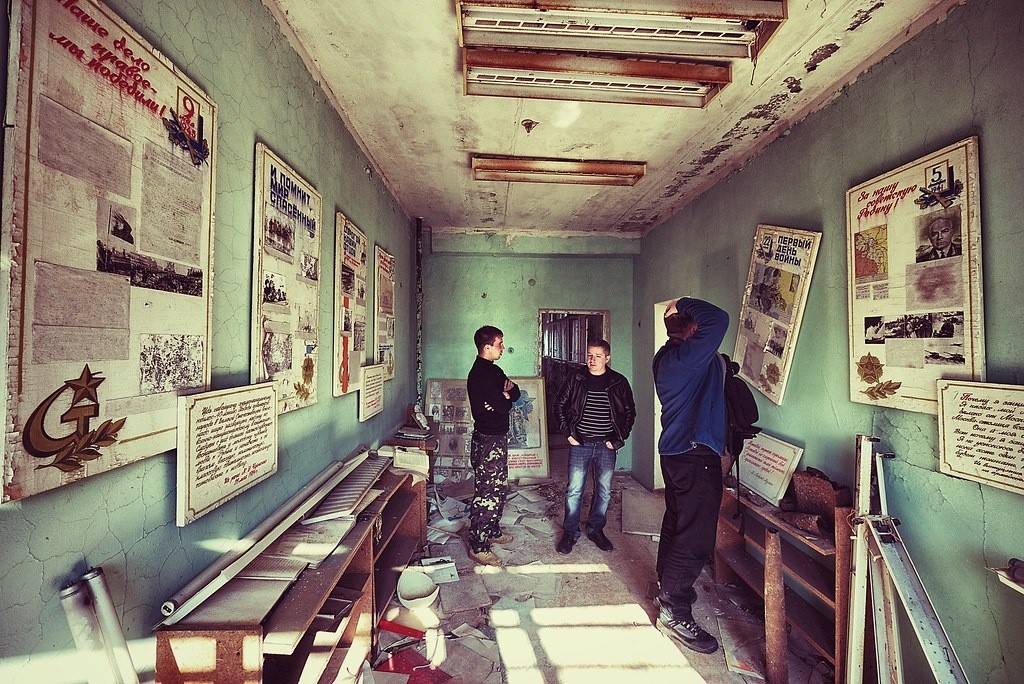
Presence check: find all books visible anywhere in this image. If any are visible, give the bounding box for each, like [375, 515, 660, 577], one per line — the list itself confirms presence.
[393, 426, 434, 441]
[235, 488, 384, 580]
[377, 445, 430, 475]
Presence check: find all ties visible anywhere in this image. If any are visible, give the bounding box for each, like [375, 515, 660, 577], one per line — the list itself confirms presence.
[940, 251, 945, 259]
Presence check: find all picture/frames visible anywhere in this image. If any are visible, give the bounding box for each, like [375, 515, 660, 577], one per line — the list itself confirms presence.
[732, 433, 805, 507]
[505, 377, 549, 481]
[375, 245, 396, 381]
[358, 365, 383, 422]
[424, 378, 475, 482]
[845, 136, 984, 415]
[0, 0, 216, 497]
[934, 378, 1024, 495]
[249, 145, 318, 416]
[329, 211, 369, 397]
[724, 224, 822, 404]
[177, 381, 282, 529]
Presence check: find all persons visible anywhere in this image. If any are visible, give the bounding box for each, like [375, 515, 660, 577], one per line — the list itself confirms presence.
[553, 340, 638, 554]
[905, 315, 933, 338]
[264, 279, 286, 302]
[431, 406, 440, 419]
[915, 218, 961, 263]
[744, 311, 753, 330]
[652, 297, 730, 653]
[466, 325, 521, 566]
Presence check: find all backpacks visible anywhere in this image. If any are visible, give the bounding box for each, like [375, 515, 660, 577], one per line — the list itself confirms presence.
[654, 343, 762, 456]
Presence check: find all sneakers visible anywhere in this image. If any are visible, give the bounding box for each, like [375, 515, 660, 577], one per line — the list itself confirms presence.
[468, 546, 502, 567]
[488, 532, 513, 544]
[557, 525, 581, 555]
[652, 578, 698, 609]
[587, 529, 613, 551]
[656, 605, 718, 654]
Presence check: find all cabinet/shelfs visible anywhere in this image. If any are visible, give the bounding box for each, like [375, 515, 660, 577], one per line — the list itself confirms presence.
[718, 479, 853, 684]
[150, 423, 440, 684]
